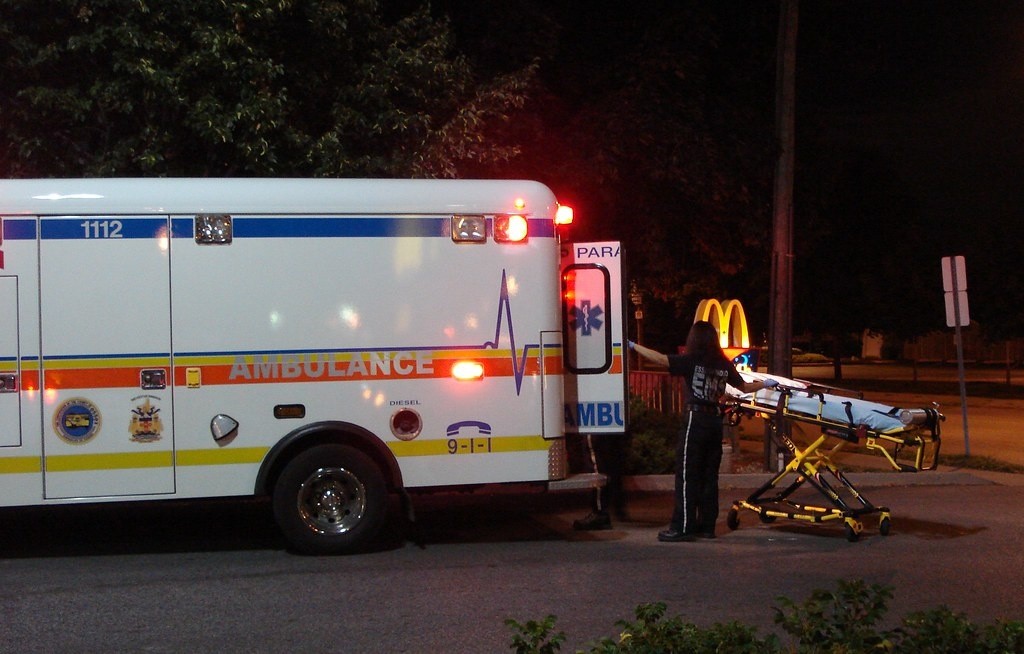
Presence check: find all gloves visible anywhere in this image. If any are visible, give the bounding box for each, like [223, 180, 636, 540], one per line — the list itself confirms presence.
[764, 379, 779, 389]
[627, 338, 635, 350]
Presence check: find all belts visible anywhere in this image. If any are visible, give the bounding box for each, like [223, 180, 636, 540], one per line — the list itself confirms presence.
[686, 403, 715, 415]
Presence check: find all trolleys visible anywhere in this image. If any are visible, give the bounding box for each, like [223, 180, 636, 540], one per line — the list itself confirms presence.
[718, 368, 948, 542]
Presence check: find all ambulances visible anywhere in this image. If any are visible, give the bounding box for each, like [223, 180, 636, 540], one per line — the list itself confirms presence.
[1, 178, 630, 556]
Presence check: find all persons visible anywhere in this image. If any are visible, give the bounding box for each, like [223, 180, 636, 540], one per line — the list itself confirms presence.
[573, 434, 628, 529]
[628, 321, 780, 542]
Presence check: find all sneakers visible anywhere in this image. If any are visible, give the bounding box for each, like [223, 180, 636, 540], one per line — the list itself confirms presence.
[700, 528, 715, 539]
[617, 511, 628, 521]
[574, 512, 612, 531]
[658, 528, 696, 542]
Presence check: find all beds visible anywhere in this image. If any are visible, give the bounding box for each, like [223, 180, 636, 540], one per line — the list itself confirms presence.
[721, 372, 947, 445]
